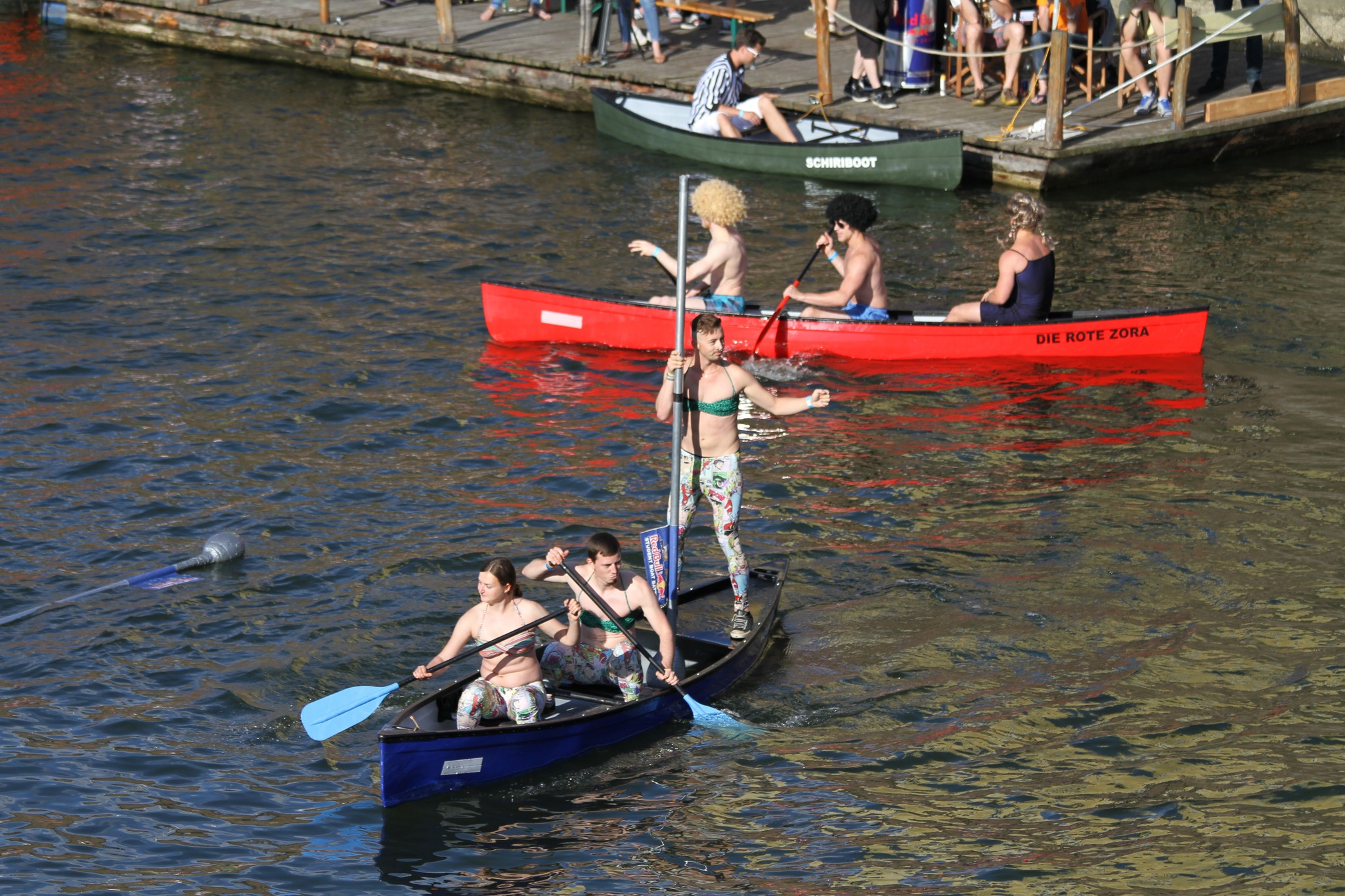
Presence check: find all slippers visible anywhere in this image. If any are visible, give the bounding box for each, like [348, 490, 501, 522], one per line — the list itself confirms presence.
[1063, 96, 1069, 106]
[1031, 94, 1047, 106]
[652, 48, 666, 63]
[617, 49, 633, 58]
[667, 11, 683, 24]
[633, 7, 644, 20]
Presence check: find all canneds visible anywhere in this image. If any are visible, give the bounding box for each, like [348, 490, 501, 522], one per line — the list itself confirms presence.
[939, 74, 946, 97]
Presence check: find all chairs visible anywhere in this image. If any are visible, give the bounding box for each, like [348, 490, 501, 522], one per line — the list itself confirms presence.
[946, 0, 1173, 111]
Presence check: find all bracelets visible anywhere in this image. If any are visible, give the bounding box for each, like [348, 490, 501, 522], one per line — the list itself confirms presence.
[828, 252, 838, 261]
[666, 373, 675, 381]
[545, 563, 555, 571]
[806, 396, 814, 409]
[653, 247, 661, 258]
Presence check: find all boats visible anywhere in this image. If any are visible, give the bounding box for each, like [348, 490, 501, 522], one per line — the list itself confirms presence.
[586, 83, 965, 192]
[375, 552, 795, 809]
[477, 278, 1210, 362]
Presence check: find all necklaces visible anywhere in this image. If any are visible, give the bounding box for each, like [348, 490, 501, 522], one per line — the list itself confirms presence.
[690, 362, 722, 378]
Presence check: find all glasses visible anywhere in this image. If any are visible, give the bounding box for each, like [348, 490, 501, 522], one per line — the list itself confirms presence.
[747, 47, 760, 58]
[829, 220, 849, 228]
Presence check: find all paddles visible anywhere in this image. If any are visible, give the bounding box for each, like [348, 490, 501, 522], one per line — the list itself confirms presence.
[300, 606, 578, 742]
[752, 223, 835, 352]
[652, 256, 677, 289]
[549, 562, 733, 719]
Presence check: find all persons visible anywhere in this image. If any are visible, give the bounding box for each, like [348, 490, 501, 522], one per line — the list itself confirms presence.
[945, 193, 1055, 322]
[482, 0, 1263, 144]
[655, 315, 829, 639]
[522, 533, 681, 703]
[783, 194, 887, 321]
[627, 180, 751, 312]
[412, 558, 583, 732]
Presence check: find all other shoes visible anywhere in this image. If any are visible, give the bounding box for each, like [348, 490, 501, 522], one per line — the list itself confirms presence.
[378, 0, 397, 7]
[804, 22, 817, 39]
[1168, 86, 1196, 101]
[646, 30, 671, 45]
[1198, 78, 1225, 94]
[629, 26, 648, 46]
[1249, 80, 1263, 94]
[718, 17, 731, 34]
[679, 13, 712, 29]
[827, 21, 844, 37]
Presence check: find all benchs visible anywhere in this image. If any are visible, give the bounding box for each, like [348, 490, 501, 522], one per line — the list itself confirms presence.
[633, 0, 775, 51]
[437, 680, 666, 728]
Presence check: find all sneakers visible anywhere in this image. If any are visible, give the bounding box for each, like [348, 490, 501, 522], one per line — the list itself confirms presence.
[859, 75, 873, 91]
[843, 84, 869, 102]
[1134, 90, 1158, 115]
[1157, 98, 1173, 118]
[971, 88, 987, 106]
[871, 91, 897, 109]
[1000, 87, 1019, 106]
[730, 609, 753, 638]
